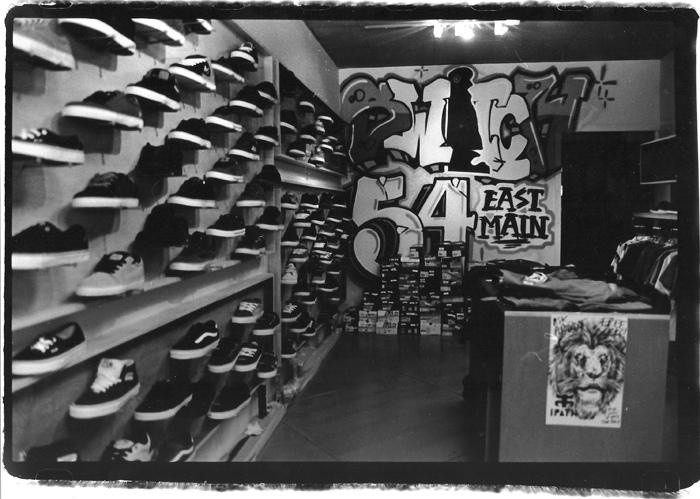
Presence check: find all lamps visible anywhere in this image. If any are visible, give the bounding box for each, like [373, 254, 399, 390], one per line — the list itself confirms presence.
[433, 19, 520, 41]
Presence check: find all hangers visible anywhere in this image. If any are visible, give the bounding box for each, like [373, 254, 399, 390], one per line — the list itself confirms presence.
[633, 219, 678, 244]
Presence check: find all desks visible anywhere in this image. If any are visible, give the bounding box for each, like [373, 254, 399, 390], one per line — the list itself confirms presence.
[467, 262, 673, 462]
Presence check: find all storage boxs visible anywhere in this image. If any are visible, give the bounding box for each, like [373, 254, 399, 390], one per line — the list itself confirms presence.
[344, 239, 467, 336]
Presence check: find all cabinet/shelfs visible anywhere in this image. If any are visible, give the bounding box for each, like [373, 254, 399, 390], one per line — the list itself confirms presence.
[274, 58, 352, 400]
[635, 133, 678, 220]
[12, 15, 285, 463]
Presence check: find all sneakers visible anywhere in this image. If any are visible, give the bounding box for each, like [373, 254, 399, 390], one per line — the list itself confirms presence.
[282, 71, 353, 359]
